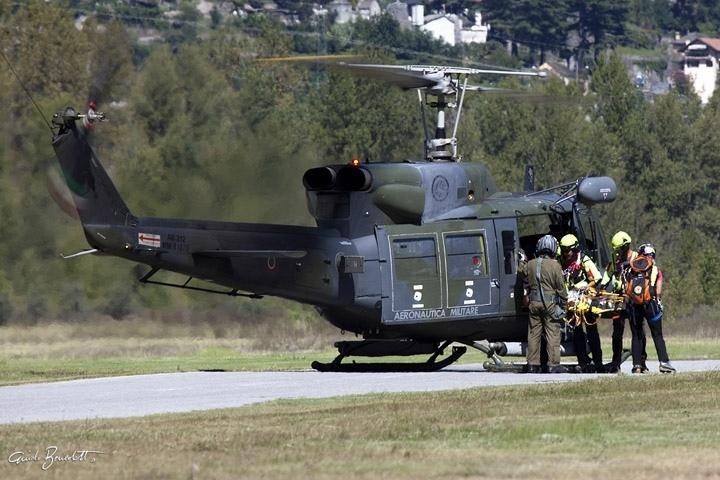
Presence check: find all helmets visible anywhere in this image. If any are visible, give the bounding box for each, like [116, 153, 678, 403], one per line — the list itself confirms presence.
[536, 235, 561, 256]
[560, 234, 579, 248]
[612, 231, 631, 250]
[638, 244, 656, 255]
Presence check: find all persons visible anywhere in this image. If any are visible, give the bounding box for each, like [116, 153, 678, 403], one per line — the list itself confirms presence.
[518, 231, 677, 373]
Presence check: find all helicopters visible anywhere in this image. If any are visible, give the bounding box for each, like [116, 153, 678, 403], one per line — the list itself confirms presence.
[49, 59, 620, 378]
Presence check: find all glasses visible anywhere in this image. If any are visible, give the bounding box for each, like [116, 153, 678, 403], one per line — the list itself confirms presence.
[562, 248, 573, 253]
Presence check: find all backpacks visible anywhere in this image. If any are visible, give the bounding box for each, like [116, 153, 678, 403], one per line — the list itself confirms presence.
[626, 256, 652, 312]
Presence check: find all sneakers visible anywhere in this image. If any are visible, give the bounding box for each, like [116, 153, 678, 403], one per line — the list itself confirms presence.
[528, 362, 676, 373]
